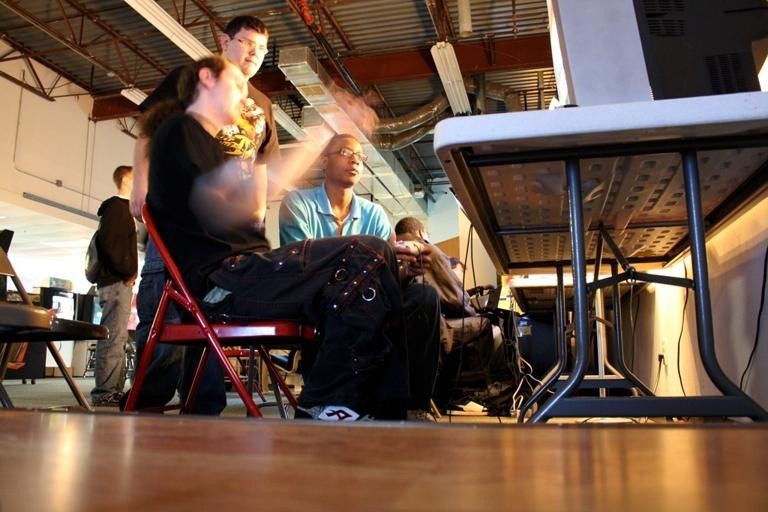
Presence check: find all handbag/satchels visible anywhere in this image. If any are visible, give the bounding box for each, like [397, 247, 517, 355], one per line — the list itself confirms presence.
[83, 231, 98, 284]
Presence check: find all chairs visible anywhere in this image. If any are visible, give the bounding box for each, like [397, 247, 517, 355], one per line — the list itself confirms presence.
[121, 203, 314, 417]
[1, 226, 111, 417]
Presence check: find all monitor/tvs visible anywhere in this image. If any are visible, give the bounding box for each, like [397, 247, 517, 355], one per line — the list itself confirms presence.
[547, 1, 762, 107]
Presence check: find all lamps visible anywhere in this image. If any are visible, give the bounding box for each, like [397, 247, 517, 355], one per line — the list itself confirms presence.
[119, 87, 149, 106]
[429, 41, 473, 117]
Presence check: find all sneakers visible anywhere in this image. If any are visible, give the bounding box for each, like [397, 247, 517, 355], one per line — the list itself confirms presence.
[447, 399, 488, 416]
[293, 406, 378, 422]
[92, 392, 126, 407]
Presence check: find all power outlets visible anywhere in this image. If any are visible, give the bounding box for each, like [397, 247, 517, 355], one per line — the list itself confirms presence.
[662, 349, 668, 365]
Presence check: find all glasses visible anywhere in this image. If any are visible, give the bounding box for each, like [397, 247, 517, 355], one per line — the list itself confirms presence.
[325, 147, 368, 162]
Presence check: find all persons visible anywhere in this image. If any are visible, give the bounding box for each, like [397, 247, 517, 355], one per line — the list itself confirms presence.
[447, 257, 495, 297]
[278, 134, 443, 423]
[88, 164, 139, 409]
[147, 56, 406, 425]
[396, 217, 517, 418]
[116, 13, 283, 414]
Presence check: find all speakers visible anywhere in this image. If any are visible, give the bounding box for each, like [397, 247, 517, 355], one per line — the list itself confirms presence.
[511, 311, 578, 412]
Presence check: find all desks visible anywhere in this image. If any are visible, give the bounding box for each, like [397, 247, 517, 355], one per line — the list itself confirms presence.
[432, 90, 767, 425]
